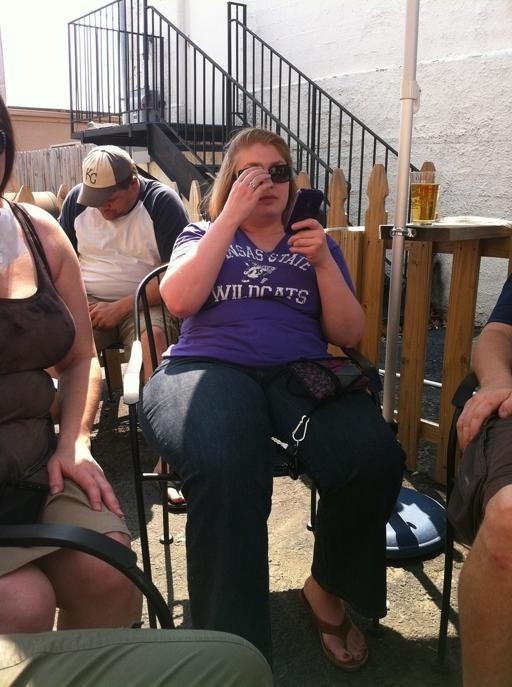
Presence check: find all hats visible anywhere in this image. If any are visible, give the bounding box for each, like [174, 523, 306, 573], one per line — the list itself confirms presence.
[76, 145, 135, 207]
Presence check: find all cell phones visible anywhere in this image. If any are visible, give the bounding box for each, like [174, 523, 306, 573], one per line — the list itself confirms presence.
[284, 187, 323, 235]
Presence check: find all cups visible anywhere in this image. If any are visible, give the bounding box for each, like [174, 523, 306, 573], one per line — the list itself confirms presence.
[409, 171, 442, 225]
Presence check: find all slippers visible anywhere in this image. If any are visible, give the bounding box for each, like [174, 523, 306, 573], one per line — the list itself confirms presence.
[153, 480, 187, 514]
[300, 587, 369, 672]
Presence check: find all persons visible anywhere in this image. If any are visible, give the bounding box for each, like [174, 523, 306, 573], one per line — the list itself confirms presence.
[0, 102, 146, 636]
[57, 145, 190, 511]
[445, 268, 511, 686]
[0, 626, 277, 687]
[139, 127, 402, 671]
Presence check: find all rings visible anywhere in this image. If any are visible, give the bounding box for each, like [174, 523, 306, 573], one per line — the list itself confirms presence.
[249, 181, 257, 188]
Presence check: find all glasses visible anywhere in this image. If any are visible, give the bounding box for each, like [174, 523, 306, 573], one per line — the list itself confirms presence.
[238, 165, 292, 183]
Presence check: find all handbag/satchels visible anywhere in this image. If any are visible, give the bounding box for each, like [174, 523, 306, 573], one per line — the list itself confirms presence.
[287, 357, 368, 409]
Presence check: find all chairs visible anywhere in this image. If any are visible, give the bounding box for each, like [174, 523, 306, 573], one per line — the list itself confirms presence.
[123, 264, 383, 628]
[0, 524, 174, 628]
[432, 372, 478, 672]
